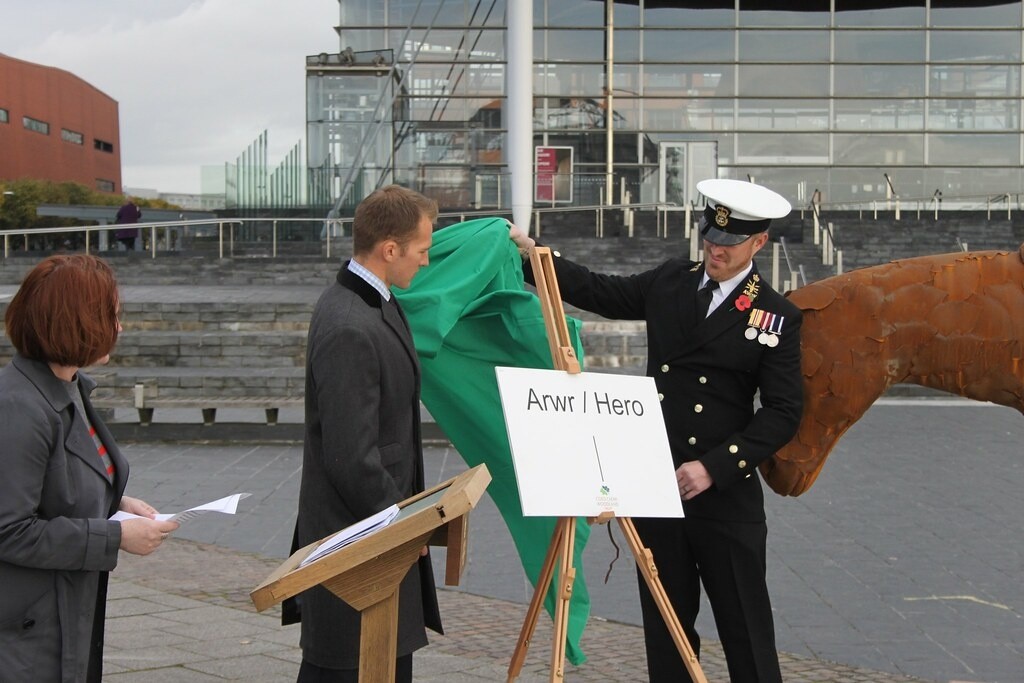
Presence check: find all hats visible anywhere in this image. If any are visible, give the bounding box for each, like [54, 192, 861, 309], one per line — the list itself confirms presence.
[697, 178, 791, 246]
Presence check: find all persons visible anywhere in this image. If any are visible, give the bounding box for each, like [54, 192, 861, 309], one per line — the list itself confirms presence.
[1, 252, 180, 683]
[494, 179, 805, 683]
[279, 185, 449, 682]
[113, 194, 142, 252]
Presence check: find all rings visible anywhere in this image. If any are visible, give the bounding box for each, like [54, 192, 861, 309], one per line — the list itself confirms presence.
[683, 487, 689, 493]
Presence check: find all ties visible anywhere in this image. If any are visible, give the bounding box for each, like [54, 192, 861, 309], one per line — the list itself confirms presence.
[696, 280, 719, 327]
